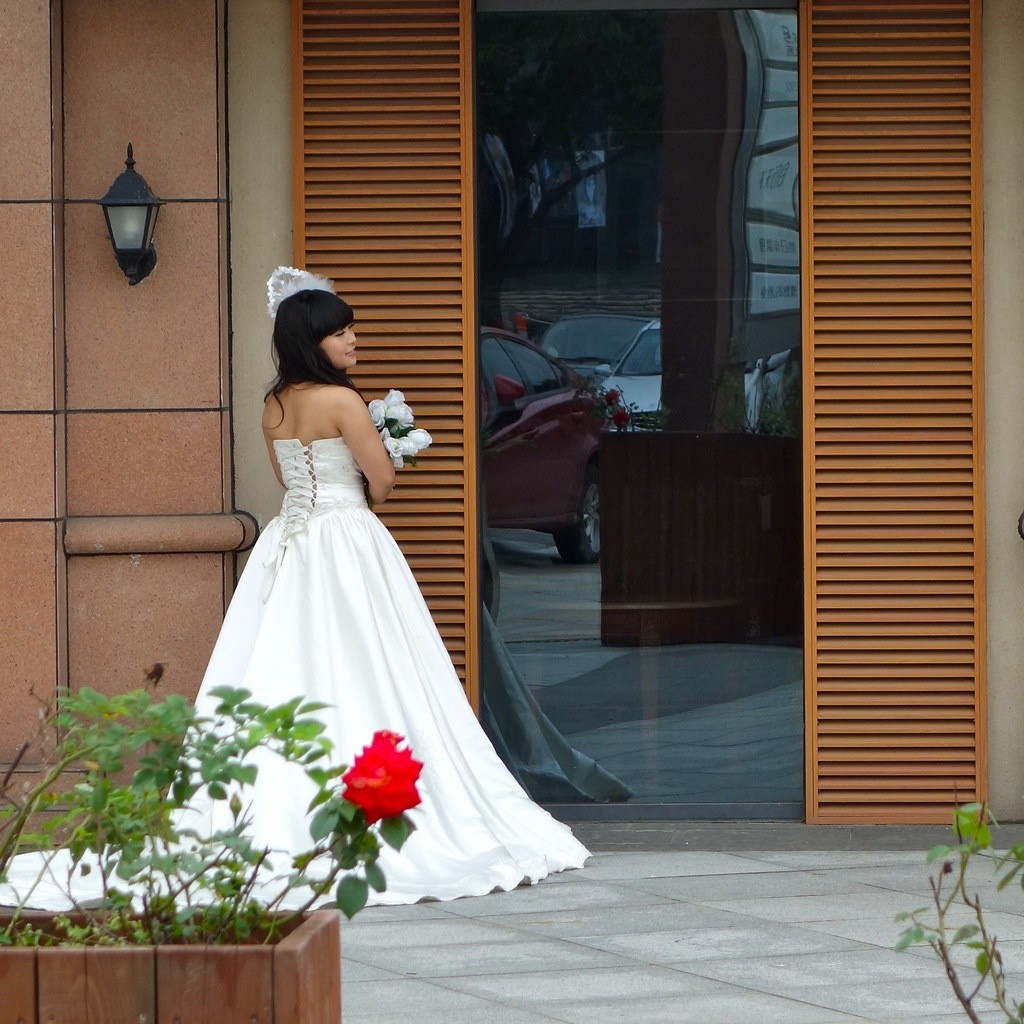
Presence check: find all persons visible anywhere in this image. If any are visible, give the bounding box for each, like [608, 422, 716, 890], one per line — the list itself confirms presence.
[0, 264, 596, 914]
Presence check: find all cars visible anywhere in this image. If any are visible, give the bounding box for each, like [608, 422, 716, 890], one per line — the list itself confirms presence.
[539, 315, 789, 433]
[478, 325, 610, 562]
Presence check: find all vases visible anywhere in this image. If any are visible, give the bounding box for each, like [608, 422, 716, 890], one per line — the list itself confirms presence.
[0, 909, 342, 1024]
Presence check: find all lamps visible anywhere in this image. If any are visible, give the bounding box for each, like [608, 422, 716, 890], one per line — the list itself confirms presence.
[96, 144, 166, 289]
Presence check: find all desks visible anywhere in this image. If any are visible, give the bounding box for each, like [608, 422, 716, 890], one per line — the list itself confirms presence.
[522, 583, 748, 797]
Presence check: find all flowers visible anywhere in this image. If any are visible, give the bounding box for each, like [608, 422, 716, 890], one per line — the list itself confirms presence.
[0, 685, 427, 942]
